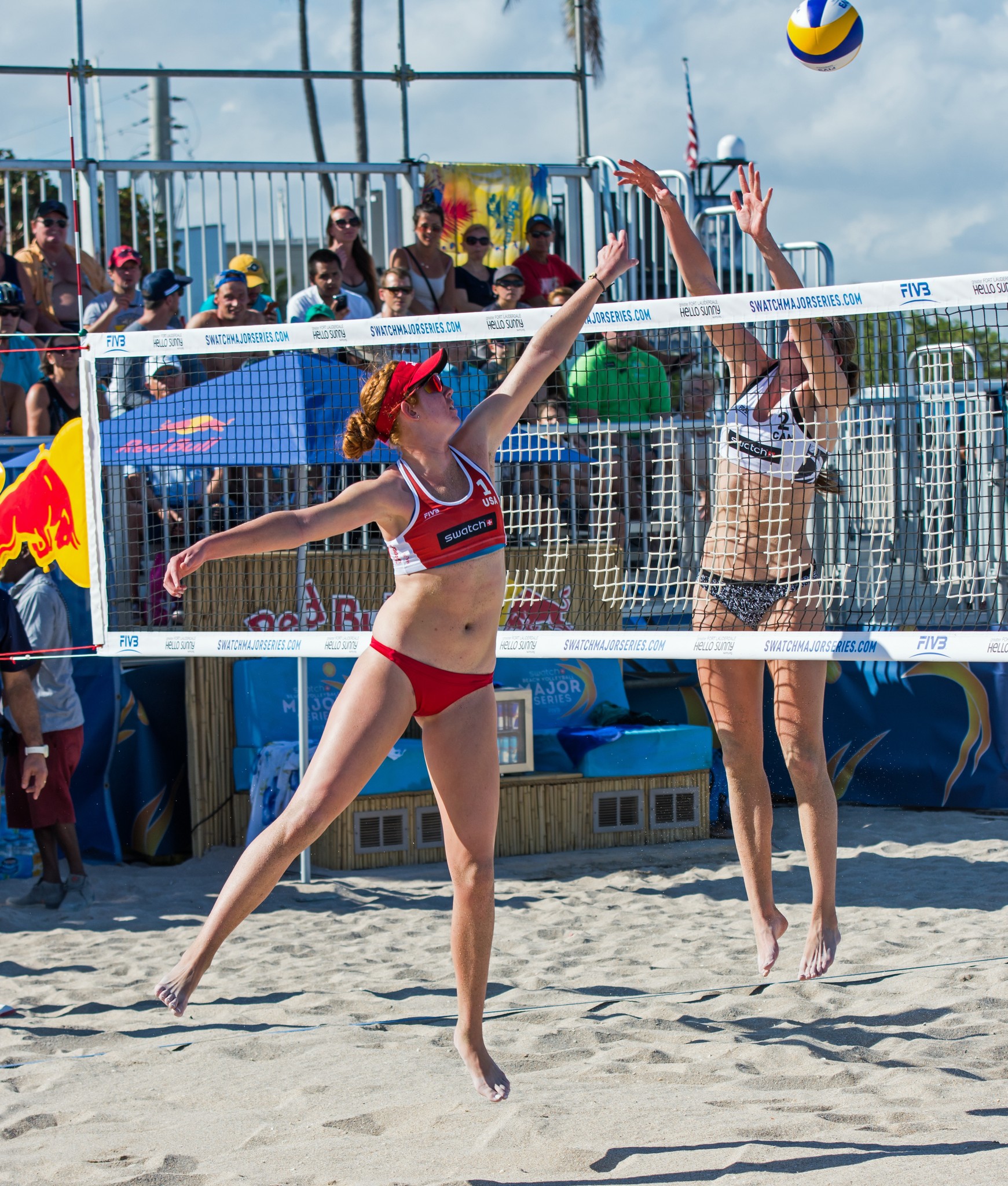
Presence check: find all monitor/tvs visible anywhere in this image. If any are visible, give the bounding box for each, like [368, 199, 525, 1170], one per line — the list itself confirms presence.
[494, 689, 536, 776]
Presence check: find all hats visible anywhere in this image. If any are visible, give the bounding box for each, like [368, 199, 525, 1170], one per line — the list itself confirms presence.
[306, 304, 336, 323]
[493, 266, 524, 283]
[108, 245, 141, 268]
[141, 268, 193, 301]
[35, 201, 68, 219]
[375, 348, 448, 444]
[213, 254, 269, 306]
[144, 355, 182, 382]
[526, 214, 552, 231]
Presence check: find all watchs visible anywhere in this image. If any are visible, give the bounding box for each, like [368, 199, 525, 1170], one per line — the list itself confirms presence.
[25, 745, 50, 758]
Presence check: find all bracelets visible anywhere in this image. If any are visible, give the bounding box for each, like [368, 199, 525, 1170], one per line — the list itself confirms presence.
[587, 273, 617, 294]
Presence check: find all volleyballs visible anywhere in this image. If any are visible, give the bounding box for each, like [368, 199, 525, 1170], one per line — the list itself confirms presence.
[786, 0, 864, 71]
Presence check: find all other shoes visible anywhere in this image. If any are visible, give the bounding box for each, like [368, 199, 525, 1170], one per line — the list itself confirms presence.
[8, 877, 65, 909]
[56, 872, 99, 909]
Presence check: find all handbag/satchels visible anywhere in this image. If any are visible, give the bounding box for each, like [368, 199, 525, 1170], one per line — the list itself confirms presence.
[151, 552, 179, 626]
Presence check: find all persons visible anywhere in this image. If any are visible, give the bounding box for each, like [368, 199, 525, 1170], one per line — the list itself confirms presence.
[156, 231, 637, 1106]
[0, 201, 718, 907]
[612, 160, 862, 979]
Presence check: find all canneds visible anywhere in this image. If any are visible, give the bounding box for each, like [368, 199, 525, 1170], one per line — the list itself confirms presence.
[496, 700, 519, 730]
[497, 735, 519, 764]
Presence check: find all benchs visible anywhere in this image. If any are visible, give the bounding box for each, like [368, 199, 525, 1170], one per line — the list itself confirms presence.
[228, 656, 712, 870]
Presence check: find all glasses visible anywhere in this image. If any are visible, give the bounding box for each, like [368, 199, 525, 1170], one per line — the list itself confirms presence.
[388, 372, 443, 418]
[415, 222, 553, 246]
[816, 317, 841, 355]
[383, 286, 413, 295]
[49, 344, 80, 354]
[494, 337, 521, 346]
[153, 369, 179, 381]
[0, 307, 22, 318]
[336, 218, 360, 229]
[118, 251, 143, 258]
[36, 219, 67, 229]
[175, 288, 184, 296]
[683, 388, 714, 399]
[492, 279, 524, 287]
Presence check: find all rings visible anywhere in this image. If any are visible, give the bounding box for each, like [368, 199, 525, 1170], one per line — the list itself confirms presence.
[44, 781, 46, 783]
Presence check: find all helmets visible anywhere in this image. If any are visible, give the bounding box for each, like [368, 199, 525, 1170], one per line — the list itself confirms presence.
[0, 281, 26, 305]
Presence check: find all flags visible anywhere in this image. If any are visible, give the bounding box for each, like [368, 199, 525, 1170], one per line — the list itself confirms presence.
[685, 66, 699, 173]
